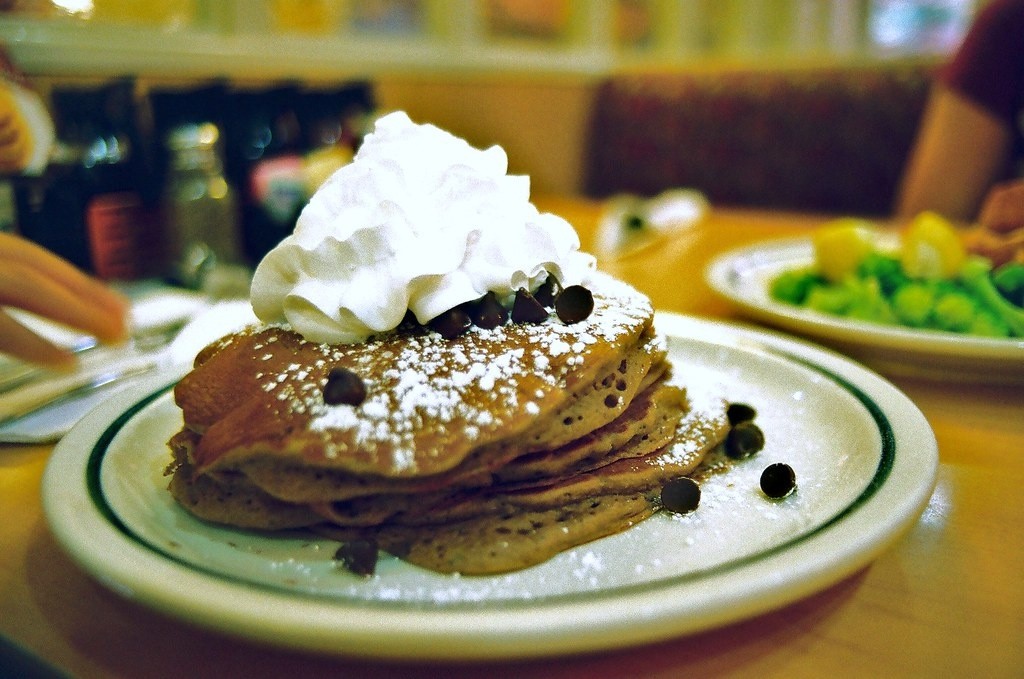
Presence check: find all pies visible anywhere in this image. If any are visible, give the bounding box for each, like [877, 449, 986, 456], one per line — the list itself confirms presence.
[162, 276, 731, 578]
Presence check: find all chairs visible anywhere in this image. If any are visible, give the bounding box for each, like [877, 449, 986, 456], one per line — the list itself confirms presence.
[581, 66, 936, 226]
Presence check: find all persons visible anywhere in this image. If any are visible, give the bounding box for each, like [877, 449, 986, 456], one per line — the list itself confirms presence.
[0, 230, 127, 369]
[891, 0, 1024, 234]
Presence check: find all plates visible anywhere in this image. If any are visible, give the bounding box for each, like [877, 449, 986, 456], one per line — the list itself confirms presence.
[702, 236, 1024, 389]
[44, 311, 939, 658]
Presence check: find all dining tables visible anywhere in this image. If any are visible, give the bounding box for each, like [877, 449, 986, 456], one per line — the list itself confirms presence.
[0, 195, 1024, 679]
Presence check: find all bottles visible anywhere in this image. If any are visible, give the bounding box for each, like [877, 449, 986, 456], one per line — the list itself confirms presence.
[19, 73, 383, 287]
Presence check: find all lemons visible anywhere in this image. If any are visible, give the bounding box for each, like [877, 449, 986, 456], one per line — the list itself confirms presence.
[810, 216, 873, 280]
[902, 208, 966, 282]
